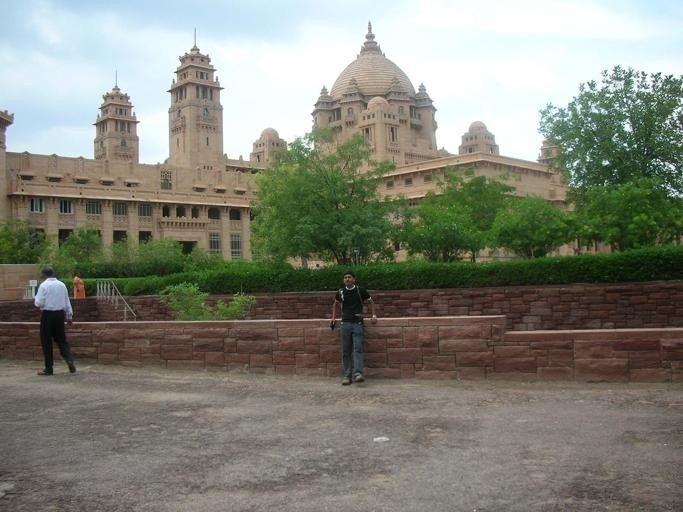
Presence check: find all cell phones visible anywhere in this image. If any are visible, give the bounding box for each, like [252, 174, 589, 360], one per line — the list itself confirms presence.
[330, 321, 335, 330]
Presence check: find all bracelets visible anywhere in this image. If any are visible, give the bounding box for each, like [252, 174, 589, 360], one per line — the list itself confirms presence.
[372, 314, 376, 318]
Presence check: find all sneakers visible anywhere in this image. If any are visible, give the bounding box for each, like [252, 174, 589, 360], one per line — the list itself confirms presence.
[355, 375, 364, 382]
[38, 370, 51, 375]
[69, 364, 76, 372]
[342, 376, 352, 385]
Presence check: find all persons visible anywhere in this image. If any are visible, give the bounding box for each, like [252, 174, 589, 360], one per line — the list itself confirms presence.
[72, 271, 86, 299]
[34, 265, 77, 376]
[330, 271, 377, 385]
